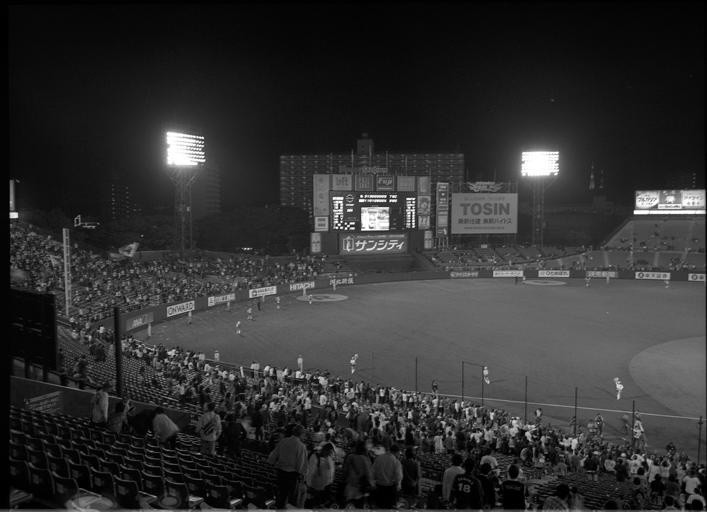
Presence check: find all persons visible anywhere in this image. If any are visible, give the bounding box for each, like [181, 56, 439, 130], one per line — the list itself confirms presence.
[4, 209, 705, 510]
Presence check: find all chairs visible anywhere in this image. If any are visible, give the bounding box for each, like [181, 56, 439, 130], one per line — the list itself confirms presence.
[7, 406, 273, 509]
